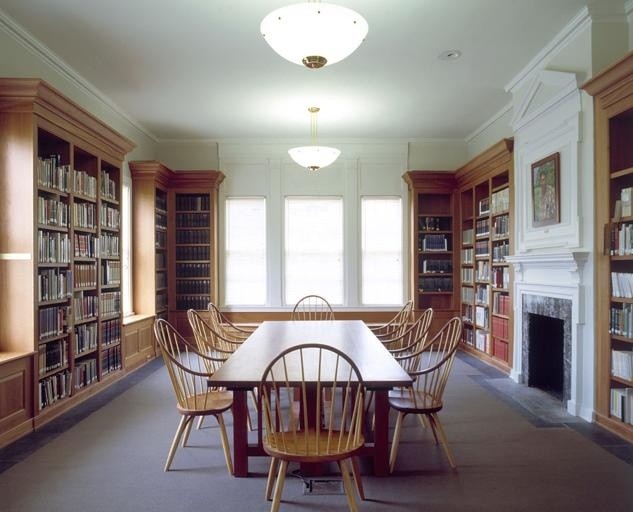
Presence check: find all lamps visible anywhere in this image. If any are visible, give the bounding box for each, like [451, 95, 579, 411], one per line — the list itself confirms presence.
[288, 107, 341, 170]
[259, 0, 368, 69]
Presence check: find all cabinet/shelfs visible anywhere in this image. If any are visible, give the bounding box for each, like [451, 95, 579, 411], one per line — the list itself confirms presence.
[129, 160, 172, 369]
[456, 135, 512, 376]
[0, 77, 135, 433]
[401, 170, 456, 352]
[581, 53, 633, 443]
[173, 170, 224, 352]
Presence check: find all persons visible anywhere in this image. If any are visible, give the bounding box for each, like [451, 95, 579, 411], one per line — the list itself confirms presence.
[539, 172, 556, 221]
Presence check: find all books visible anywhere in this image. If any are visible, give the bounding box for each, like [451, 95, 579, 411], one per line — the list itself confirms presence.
[458, 186, 510, 362]
[608, 187, 632, 427]
[156, 186, 210, 347]
[37, 153, 122, 410]
[418, 216, 453, 292]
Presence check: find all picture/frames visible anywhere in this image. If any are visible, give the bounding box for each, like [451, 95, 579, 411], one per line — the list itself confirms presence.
[531, 152, 560, 228]
